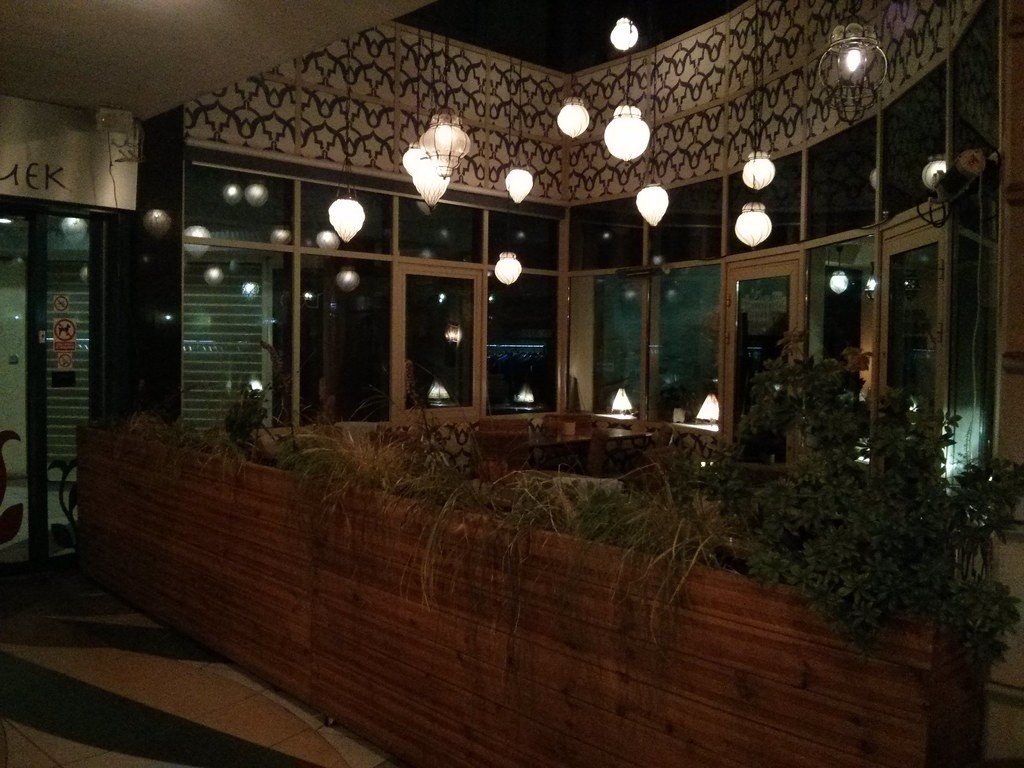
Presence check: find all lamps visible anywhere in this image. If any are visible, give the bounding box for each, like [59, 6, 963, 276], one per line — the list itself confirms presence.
[611, 382, 632, 415]
[905, 269, 923, 302]
[140, 177, 362, 303]
[830, 246, 848, 294]
[494, 53, 535, 286]
[555, 62, 589, 138]
[863, 263, 875, 300]
[734, 0, 777, 249]
[604, 1, 670, 229]
[401, 11, 473, 212]
[429, 381, 448, 406]
[328, 41, 367, 243]
[914, 152, 950, 229]
[694, 389, 720, 427]
[818, 0, 889, 126]
[515, 383, 534, 404]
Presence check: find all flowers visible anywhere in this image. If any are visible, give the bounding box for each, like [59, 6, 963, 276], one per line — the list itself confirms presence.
[76, 305, 1024, 701]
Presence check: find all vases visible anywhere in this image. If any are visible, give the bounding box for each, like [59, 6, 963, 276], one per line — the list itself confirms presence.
[72, 429, 992, 768]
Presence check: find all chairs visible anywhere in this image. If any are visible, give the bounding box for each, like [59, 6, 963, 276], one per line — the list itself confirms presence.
[471, 411, 672, 480]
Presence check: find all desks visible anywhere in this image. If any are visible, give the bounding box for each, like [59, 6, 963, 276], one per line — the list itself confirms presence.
[527, 425, 647, 474]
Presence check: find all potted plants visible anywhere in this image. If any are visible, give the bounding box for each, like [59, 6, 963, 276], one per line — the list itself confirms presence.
[659, 383, 694, 425]
